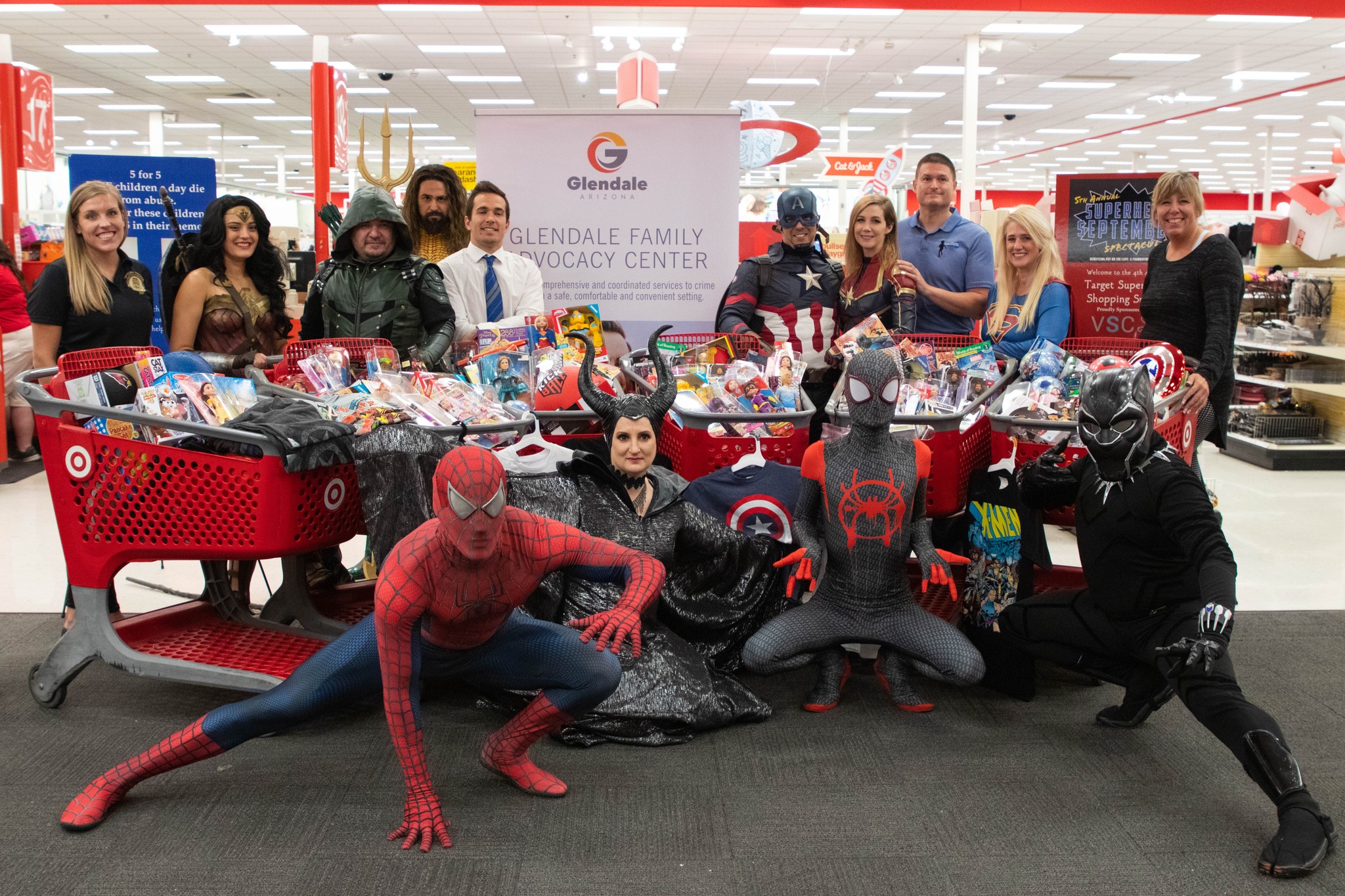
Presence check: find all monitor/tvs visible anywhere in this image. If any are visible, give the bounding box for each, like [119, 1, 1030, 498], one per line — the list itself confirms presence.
[288, 251, 316, 292]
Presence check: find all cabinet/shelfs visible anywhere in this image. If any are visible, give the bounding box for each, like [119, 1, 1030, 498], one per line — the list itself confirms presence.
[1220, 242, 1345, 470]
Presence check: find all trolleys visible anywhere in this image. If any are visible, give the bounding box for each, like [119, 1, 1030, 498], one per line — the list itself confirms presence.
[10, 343, 339, 711]
[244, 334, 1201, 620]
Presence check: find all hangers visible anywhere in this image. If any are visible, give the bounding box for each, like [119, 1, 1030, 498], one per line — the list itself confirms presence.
[731, 433, 766, 473]
[988, 436, 1018, 488]
[497, 411, 574, 474]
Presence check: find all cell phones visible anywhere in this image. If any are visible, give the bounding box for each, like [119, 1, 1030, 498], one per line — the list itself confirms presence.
[814, 234, 824, 257]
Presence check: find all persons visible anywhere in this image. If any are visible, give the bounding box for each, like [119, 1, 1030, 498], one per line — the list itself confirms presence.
[714, 187, 844, 446]
[980, 205, 1072, 379]
[1139, 169, 1244, 526]
[437, 181, 544, 341]
[60, 445, 666, 852]
[533, 316, 556, 350]
[400, 164, 471, 264]
[0, 238, 40, 461]
[199, 349, 506, 442]
[298, 187, 457, 579]
[895, 364, 1072, 426]
[169, 194, 294, 617]
[743, 351, 986, 709]
[988, 364, 1332, 877]
[503, 326, 790, 745]
[897, 153, 994, 348]
[602, 320, 632, 392]
[26, 180, 157, 637]
[825, 195, 916, 388]
[675, 356, 800, 415]
[491, 356, 521, 402]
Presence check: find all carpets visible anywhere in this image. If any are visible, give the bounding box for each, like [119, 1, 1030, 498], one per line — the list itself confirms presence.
[0, 610, 1345, 896]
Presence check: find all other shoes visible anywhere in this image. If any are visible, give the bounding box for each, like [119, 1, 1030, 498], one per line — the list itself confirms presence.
[9, 446, 45, 462]
[60, 604, 84, 640]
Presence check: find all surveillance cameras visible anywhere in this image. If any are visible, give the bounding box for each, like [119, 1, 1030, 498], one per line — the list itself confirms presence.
[378, 72, 393, 81]
[294, 170, 299, 172]
[776, 179, 779, 182]
[1004, 114, 1016, 120]
[1311, 167, 1314, 169]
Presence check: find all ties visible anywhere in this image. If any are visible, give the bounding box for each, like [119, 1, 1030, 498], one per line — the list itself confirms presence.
[482, 253, 505, 322]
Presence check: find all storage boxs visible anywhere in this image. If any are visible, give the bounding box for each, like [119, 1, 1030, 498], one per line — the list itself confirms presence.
[1251, 173, 1345, 260]
[63, 304, 1091, 447]
